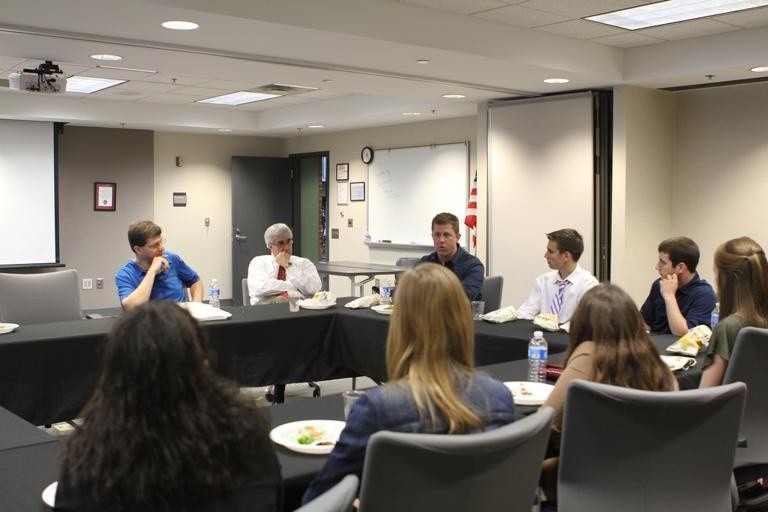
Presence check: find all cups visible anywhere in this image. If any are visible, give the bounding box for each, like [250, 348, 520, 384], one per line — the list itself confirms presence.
[471, 300, 485, 320]
[342, 391, 367, 421]
[288, 294, 300, 313]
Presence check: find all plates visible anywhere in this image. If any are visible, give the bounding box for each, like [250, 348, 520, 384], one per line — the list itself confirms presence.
[0, 322, 20, 334]
[371, 305, 394, 315]
[268, 418, 347, 455]
[296, 298, 336, 309]
[41, 481, 59, 508]
[502, 381, 555, 406]
[344, 293, 379, 310]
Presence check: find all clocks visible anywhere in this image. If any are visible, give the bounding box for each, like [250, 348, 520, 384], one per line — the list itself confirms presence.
[362, 147, 372, 163]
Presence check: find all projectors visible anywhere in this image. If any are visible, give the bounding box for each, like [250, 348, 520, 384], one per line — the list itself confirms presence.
[8, 66, 68, 93]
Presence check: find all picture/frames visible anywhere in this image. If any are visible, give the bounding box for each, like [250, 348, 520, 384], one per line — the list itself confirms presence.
[350, 181, 365, 201]
[337, 164, 350, 181]
[94, 181, 116, 212]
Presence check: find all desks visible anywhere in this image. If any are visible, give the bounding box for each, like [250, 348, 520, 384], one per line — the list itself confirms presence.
[315, 259, 405, 297]
[1, 294, 709, 512]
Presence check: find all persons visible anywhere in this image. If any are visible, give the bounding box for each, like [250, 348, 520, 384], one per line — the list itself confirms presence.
[698, 237, 767, 389]
[639, 237, 716, 336]
[515, 229, 599, 330]
[247, 223, 322, 304]
[304, 262, 515, 501]
[539, 283, 679, 495]
[115, 220, 204, 311]
[53, 302, 283, 512]
[415, 213, 484, 304]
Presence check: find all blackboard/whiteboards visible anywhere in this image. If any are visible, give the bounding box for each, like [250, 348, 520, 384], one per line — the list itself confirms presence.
[362, 140, 471, 254]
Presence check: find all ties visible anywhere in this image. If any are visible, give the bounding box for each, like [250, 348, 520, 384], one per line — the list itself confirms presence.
[549, 280, 573, 315]
[277, 265, 288, 298]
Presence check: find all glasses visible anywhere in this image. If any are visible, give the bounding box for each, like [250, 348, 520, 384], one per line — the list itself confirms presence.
[143, 239, 164, 249]
[272, 238, 294, 246]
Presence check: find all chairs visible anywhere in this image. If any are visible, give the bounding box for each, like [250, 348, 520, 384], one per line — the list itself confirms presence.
[294, 474, 360, 512]
[395, 257, 419, 280]
[480, 276, 504, 314]
[557, 380, 749, 511]
[0, 270, 81, 324]
[718, 324, 768, 507]
[353, 408, 556, 512]
[242, 278, 321, 401]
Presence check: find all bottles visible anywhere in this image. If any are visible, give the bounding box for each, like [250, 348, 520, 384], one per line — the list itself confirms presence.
[208, 279, 220, 308]
[379, 278, 390, 305]
[527, 331, 548, 384]
[711, 302, 720, 329]
